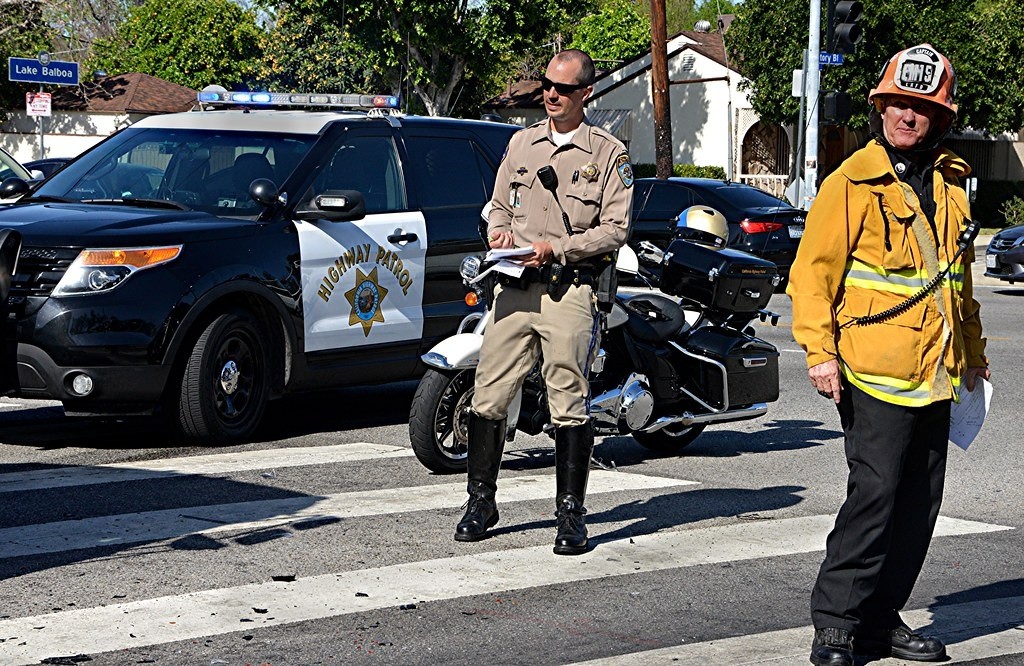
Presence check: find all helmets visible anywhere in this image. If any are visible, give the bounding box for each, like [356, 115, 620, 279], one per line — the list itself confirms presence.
[869, 43, 959, 117]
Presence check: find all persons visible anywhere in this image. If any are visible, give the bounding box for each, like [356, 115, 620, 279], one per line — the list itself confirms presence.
[785, 43, 991, 666]
[455, 48, 634, 555]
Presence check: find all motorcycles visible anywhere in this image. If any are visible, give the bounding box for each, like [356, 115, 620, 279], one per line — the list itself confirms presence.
[408, 199, 784, 476]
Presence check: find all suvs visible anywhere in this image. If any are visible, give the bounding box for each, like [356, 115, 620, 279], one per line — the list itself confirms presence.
[0, 84, 525, 447]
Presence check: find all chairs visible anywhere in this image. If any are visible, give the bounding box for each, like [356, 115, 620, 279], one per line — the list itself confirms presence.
[272, 139, 314, 198]
[319, 147, 387, 213]
[213, 153, 273, 212]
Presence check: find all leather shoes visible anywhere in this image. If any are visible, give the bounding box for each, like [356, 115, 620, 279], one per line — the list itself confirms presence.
[866, 618, 945, 661]
[810, 628, 856, 666]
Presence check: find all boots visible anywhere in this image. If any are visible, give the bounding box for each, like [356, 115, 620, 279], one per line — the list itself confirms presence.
[554, 423, 591, 555]
[454, 410, 507, 542]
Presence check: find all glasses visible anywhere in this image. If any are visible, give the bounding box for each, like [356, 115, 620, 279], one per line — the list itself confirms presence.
[541, 76, 587, 96]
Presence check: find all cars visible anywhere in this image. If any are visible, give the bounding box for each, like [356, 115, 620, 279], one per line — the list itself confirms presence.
[0, 157, 165, 201]
[984, 224, 1024, 284]
[624, 175, 809, 293]
